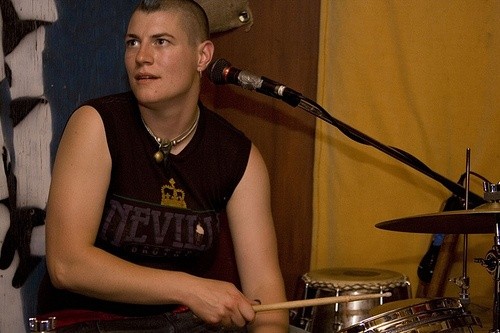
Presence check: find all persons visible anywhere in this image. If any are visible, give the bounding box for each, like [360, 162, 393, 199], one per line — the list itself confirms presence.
[36, 0, 289, 333]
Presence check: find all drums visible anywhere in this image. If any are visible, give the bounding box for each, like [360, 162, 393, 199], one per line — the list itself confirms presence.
[298, 268, 483, 333]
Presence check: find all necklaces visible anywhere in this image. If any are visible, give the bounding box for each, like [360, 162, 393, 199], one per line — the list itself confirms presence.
[138, 105, 200, 161]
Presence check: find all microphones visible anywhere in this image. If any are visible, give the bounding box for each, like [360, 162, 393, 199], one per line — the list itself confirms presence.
[206, 58, 300, 99]
[418, 174, 465, 283]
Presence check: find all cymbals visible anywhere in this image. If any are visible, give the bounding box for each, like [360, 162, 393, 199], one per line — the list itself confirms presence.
[375, 202, 500, 234]
